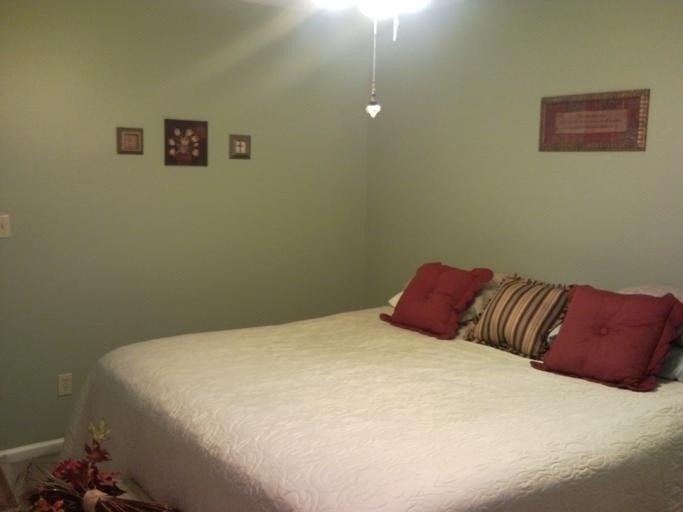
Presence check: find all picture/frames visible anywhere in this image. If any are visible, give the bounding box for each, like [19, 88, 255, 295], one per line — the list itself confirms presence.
[537, 88, 649, 151]
[116, 117, 251, 166]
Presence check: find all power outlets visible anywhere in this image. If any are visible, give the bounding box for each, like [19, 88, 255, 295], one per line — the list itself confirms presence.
[56, 374, 73, 398]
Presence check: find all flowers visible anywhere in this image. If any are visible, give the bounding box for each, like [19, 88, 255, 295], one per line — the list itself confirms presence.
[8, 418, 174, 511]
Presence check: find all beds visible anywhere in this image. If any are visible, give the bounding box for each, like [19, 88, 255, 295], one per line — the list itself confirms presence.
[77, 306, 683, 512]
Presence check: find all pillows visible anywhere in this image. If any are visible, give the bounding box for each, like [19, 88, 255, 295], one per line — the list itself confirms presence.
[533, 285, 682, 392]
[375, 260, 494, 340]
[465, 274, 573, 362]
